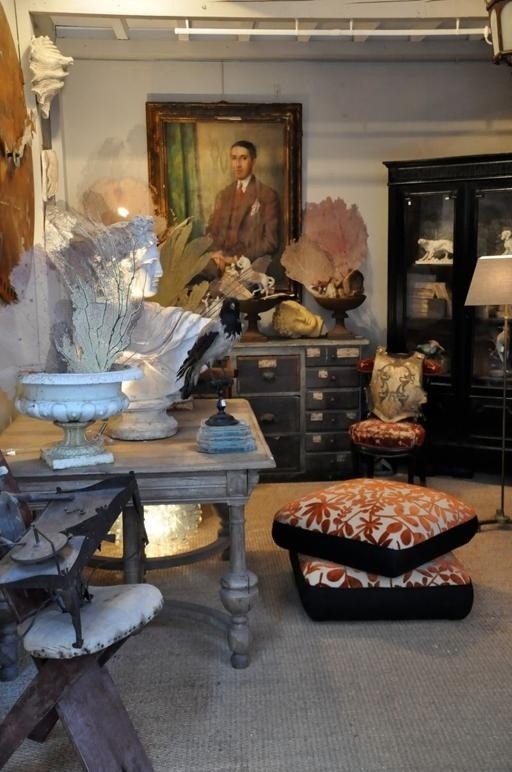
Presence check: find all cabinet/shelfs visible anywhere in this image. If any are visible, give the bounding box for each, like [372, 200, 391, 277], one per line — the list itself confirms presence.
[381, 154, 512, 475]
[228, 338, 369, 481]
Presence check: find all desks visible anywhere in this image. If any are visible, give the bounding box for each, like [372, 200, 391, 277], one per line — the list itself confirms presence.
[0, 400, 277, 668]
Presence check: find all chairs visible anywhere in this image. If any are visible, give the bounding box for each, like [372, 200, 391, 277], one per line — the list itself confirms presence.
[346, 350, 430, 484]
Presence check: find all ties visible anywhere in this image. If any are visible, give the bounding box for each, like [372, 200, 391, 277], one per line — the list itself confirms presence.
[226, 184, 244, 247]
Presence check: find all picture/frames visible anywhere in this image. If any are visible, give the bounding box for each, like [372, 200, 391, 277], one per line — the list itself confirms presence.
[145, 101, 301, 306]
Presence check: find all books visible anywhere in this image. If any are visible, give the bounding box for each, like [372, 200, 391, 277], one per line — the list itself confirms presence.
[409, 274, 452, 319]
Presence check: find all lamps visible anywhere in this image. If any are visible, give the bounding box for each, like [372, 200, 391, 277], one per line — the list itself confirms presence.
[464, 257, 512, 535]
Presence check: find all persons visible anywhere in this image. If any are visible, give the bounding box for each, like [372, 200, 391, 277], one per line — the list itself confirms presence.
[111, 217, 162, 298]
[205, 140, 280, 263]
[273, 300, 323, 338]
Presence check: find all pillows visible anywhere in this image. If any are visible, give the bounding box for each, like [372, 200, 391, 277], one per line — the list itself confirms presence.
[290, 554, 476, 623]
[271, 478, 478, 577]
